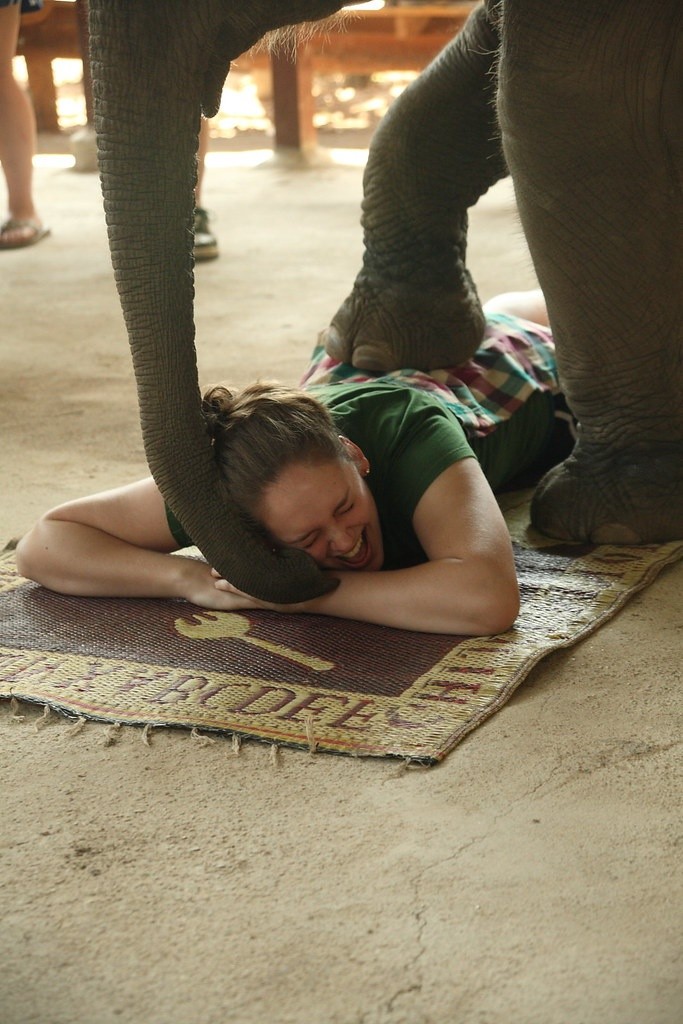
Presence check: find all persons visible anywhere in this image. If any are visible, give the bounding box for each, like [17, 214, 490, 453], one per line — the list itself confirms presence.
[193, 117, 220, 262]
[0, 0, 50, 253]
[15, 289, 579, 637]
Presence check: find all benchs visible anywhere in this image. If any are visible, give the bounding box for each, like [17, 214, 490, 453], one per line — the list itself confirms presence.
[16, 4, 475, 146]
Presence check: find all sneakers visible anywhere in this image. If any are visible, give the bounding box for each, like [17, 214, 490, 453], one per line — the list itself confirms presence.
[194, 209, 218, 258]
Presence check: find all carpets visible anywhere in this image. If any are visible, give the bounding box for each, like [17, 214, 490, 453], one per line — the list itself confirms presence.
[0, 486, 683, 770]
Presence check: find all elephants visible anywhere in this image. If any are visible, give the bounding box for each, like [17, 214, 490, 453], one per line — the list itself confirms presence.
[87, 0, 683, 604]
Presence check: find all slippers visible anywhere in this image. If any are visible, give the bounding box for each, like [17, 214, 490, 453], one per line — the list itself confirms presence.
[0, 219, 49, 247]
